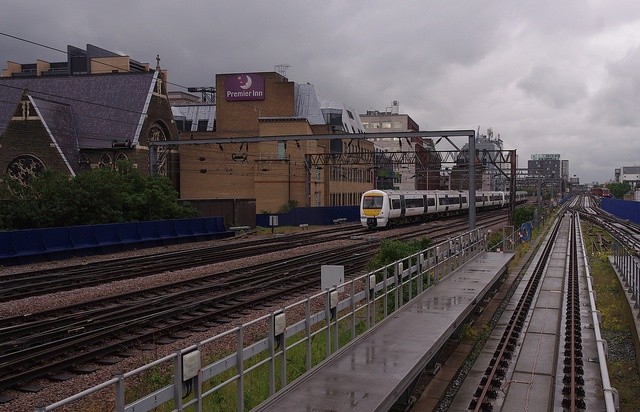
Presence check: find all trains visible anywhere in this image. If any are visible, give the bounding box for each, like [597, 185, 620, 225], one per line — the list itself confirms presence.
[360, 189, 529, 230]
